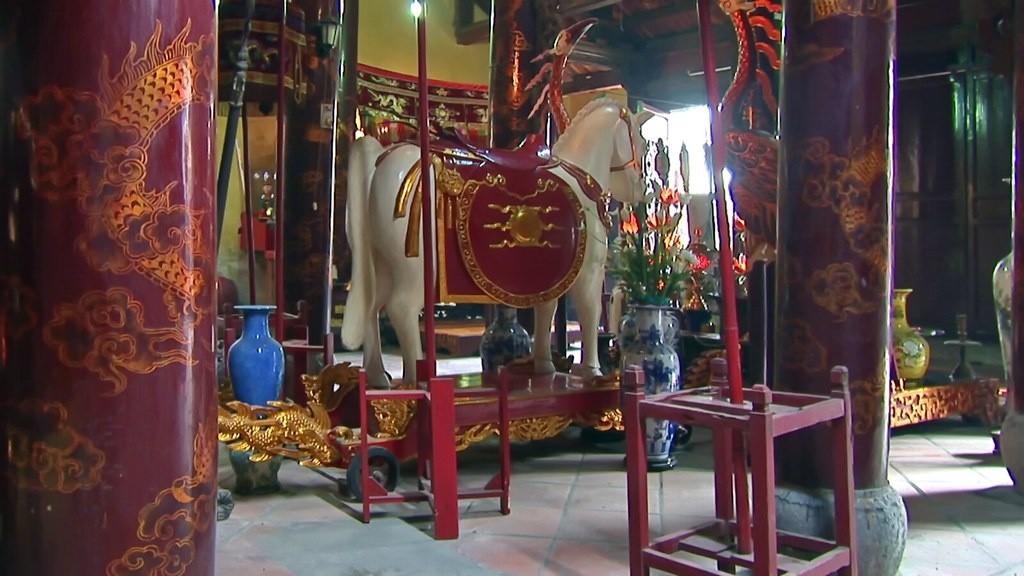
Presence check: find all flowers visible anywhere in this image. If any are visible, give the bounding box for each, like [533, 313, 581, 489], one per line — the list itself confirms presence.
[581, 181, 696, 309]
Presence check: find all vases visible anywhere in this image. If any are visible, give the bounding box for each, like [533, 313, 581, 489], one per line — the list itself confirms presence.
[228, 304, 287, 494]
[480, 302, 531, 372]
[617, 298, 681, 470]
[892, 287, 928, 378]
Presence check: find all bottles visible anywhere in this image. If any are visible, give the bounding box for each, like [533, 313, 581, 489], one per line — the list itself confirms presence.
[891, 288, 930, 388]
[228, 305, 286, 419]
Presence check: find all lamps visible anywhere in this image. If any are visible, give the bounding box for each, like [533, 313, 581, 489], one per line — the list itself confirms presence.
[317, 16, 343, 56]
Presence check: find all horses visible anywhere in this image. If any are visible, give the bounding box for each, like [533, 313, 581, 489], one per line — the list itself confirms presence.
[341, 99, 656, 390]
